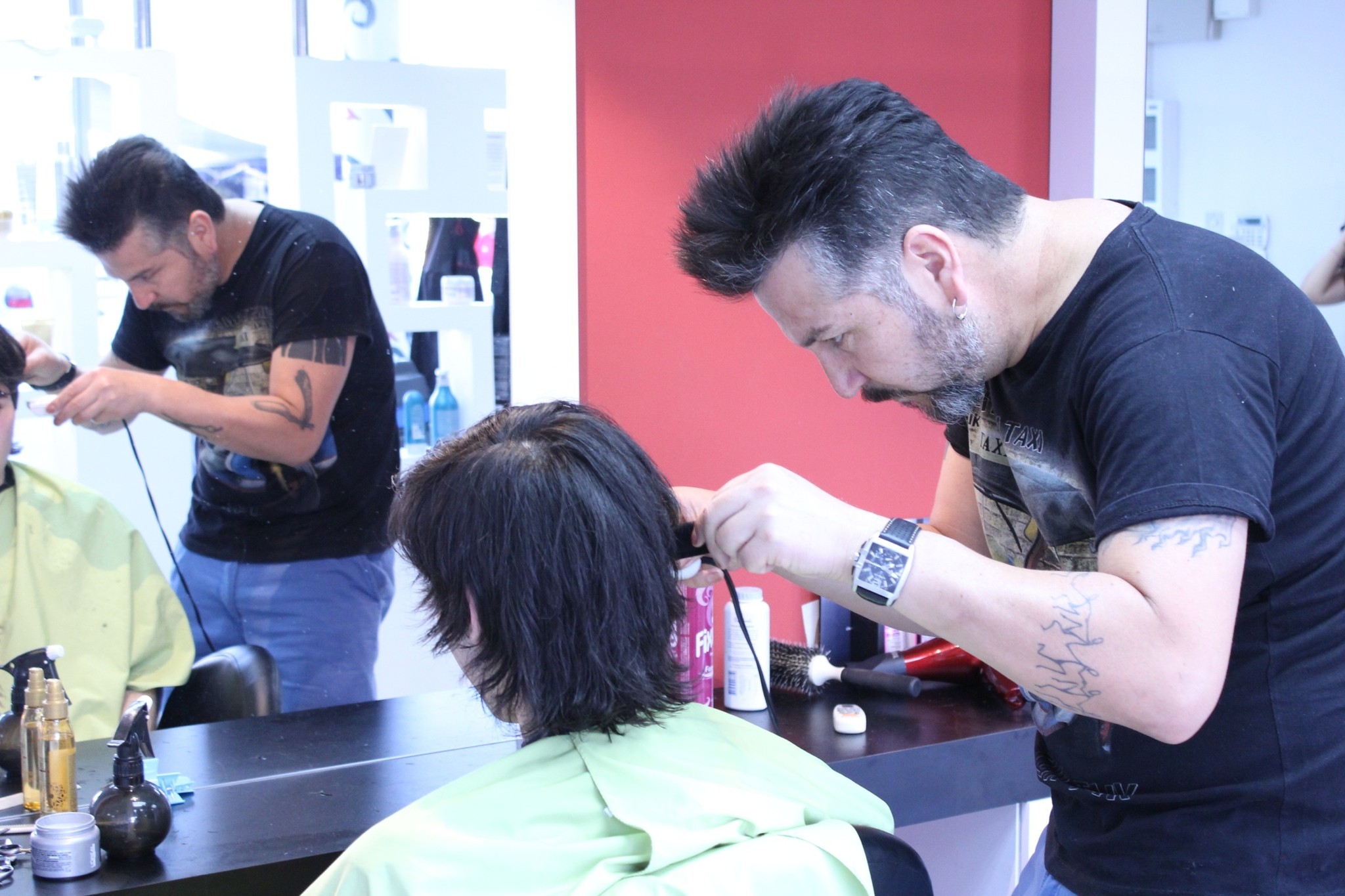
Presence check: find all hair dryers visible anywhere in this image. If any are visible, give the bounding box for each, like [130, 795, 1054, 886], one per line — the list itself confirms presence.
[840, 636, 1040, 708]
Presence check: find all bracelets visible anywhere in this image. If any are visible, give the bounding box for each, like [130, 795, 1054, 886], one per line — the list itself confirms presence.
[28, 353, 77, 392]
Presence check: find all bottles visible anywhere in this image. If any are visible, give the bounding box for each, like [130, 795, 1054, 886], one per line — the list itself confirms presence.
[429, 366, 459, 447]
[723, 586, 770, 711]
[669, 580, 714, 707]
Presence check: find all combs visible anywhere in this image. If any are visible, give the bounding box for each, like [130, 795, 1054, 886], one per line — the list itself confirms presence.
[770, 640, 923, 698]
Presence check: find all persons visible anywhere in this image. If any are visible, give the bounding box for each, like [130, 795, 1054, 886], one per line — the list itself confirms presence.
[1302, 225, 1345, 306]
[668, 80, 1345, 896]
[299, 400, 896, 896]
[0, 325, 196, 745]
[7, 136, 401, 715]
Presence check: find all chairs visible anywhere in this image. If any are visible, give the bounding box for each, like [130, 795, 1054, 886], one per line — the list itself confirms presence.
[155, 644, 284, 730]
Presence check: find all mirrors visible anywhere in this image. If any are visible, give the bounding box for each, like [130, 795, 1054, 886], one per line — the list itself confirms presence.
[1142, 0, 1345, 356]
[0, 0, 582, 749]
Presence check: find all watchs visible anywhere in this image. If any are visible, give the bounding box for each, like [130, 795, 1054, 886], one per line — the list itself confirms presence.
[851, 518, 923, 609]
